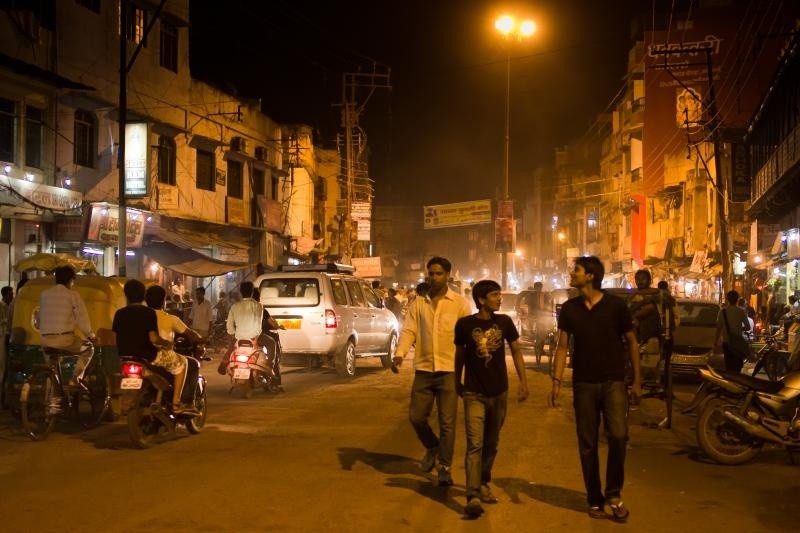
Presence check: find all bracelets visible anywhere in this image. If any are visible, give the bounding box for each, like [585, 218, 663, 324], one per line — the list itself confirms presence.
[553, 378, 561, 385]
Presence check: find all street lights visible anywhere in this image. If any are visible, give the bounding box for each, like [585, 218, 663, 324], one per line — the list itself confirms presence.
[494, 13, 538, 291]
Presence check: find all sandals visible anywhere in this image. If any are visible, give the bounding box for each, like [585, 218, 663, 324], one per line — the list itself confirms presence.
[171, 402, 185, 415]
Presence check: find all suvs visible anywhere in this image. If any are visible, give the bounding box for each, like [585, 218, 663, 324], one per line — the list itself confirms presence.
[252, 262, 400, 377]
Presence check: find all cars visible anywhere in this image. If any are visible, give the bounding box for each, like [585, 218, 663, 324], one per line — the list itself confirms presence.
[502, 288, 634, 349]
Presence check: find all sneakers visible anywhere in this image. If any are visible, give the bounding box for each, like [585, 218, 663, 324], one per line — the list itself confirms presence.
[437, 464, 453, 485]
[72, 377, 88, 390]
[421, 449, 435, 471]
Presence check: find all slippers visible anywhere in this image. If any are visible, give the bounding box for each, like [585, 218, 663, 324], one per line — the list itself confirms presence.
[589, 506, 608, 518]
[479, 491, 498, 502]
[465, 502, 484, 517]
[610, 501, 630, 518]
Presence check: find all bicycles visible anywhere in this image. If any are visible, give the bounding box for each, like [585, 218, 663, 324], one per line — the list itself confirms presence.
[534, 327, 558, 380]
[744, 325, 787, 384]
[19, 336, 111, 442]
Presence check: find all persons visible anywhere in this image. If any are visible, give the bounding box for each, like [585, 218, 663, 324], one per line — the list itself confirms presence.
[453, 279, 528, 516]
[633, 269, 660, 339]
[547, 255, 643, 519]
[644, 280, 681, 400]
[0, 278, 29, 334]
[530, 283, 552, 336]
[371, 277, 476, 316]
[224, 280, 285, 392]
[391, 257, 472, 486]
[163, 276, 240, 344]
[38, 267, 95, 395]
[711, 287, 799, 374]
[113, 278, 202, 414]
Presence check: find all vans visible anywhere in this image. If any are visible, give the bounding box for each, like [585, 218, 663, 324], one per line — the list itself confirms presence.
[668, 299, 720, 384]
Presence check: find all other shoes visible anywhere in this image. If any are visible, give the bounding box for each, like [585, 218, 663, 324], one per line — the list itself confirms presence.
[218, 360, 228, 374]
[183, 406, 202, 416]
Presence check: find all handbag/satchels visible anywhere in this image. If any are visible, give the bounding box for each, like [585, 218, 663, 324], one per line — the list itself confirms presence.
[728, 336, 750, 357]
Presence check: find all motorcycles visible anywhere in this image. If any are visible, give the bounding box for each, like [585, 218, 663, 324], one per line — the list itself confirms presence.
[5, 274, 126, 399]
[118, 339, 213, 449]
[682, 360, 800, 467]
[226, 326, 281, 399]
